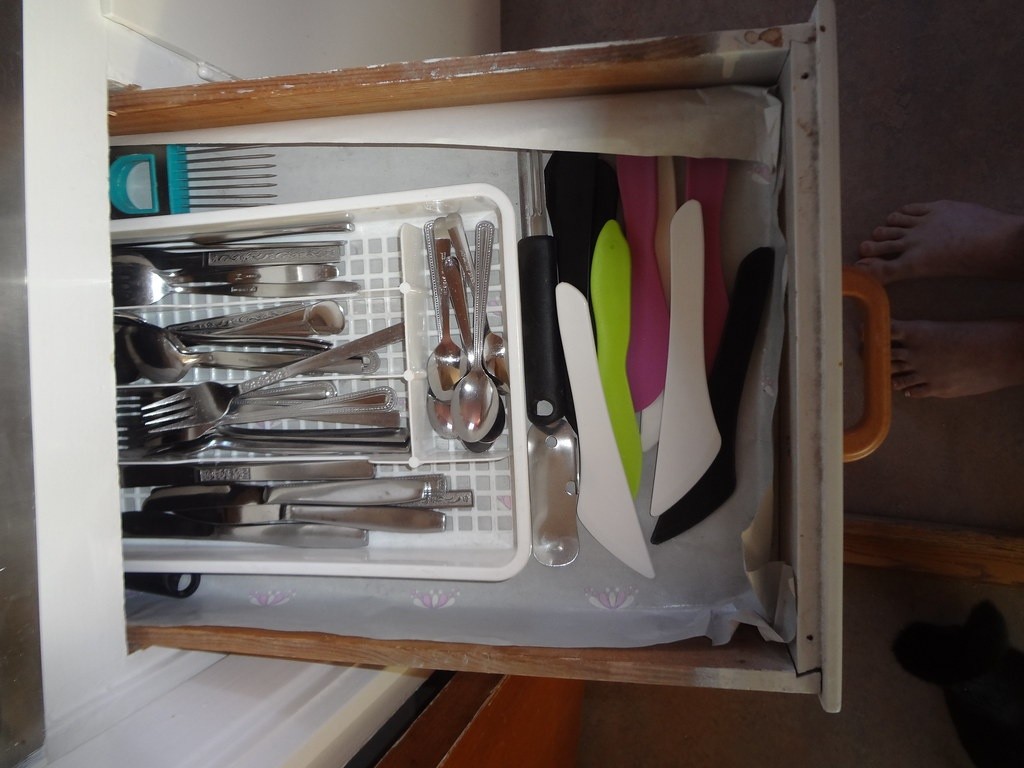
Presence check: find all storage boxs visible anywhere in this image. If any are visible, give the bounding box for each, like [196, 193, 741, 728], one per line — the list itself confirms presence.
[108, 185, 535, 583]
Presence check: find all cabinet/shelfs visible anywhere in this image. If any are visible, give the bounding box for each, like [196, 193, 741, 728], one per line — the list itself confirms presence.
[15, 0, 898, 768]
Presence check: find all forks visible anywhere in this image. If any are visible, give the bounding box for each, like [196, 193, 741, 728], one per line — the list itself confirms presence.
[116, 321, 410, 459]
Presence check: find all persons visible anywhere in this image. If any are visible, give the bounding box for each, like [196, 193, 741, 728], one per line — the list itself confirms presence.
[855, 198, 1024, 399]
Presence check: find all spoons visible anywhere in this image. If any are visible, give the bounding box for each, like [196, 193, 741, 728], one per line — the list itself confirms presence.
[423, 211, 510, 454]
[111, 223, 381, 384]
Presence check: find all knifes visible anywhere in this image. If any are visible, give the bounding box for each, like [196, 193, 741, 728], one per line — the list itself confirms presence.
[120, 462, 474, 551]
